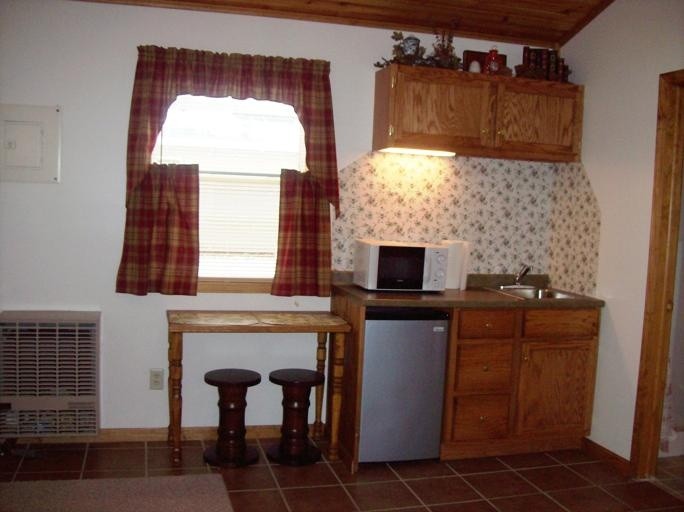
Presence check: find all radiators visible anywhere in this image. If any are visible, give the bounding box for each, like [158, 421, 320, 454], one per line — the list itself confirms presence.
[0, 311, 101, 438]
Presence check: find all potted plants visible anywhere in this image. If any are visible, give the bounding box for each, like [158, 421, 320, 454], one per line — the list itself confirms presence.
[427, 27, 461, 70]
[374, 31, 415, 68]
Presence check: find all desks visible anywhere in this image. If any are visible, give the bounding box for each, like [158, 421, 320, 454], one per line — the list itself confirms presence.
[166, 310, 352, 467]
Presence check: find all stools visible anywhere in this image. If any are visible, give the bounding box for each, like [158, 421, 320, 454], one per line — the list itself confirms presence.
[266, 368, 325, 465]
[203, 368, 261, 467]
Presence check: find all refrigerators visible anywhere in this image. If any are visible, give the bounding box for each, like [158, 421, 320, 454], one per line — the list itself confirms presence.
[359, 312, 450, 463]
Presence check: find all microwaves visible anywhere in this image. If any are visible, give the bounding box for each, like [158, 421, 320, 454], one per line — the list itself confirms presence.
[353, 237, 447, 294]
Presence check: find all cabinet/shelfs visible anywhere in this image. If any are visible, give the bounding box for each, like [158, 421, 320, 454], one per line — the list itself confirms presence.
[439, 308, 601, 461]
[373, 65, 585, 163]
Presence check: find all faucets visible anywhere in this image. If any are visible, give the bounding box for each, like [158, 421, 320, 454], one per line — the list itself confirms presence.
[514, 265, 531, 282]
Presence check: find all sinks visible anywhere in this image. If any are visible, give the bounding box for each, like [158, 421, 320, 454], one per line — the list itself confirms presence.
[496, 283, 576, 301]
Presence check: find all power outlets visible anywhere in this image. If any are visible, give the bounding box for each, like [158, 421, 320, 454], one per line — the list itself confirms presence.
[150, 369, 164, 390]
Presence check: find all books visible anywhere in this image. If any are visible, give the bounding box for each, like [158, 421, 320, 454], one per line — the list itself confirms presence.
[522, 45, 571, 82]
